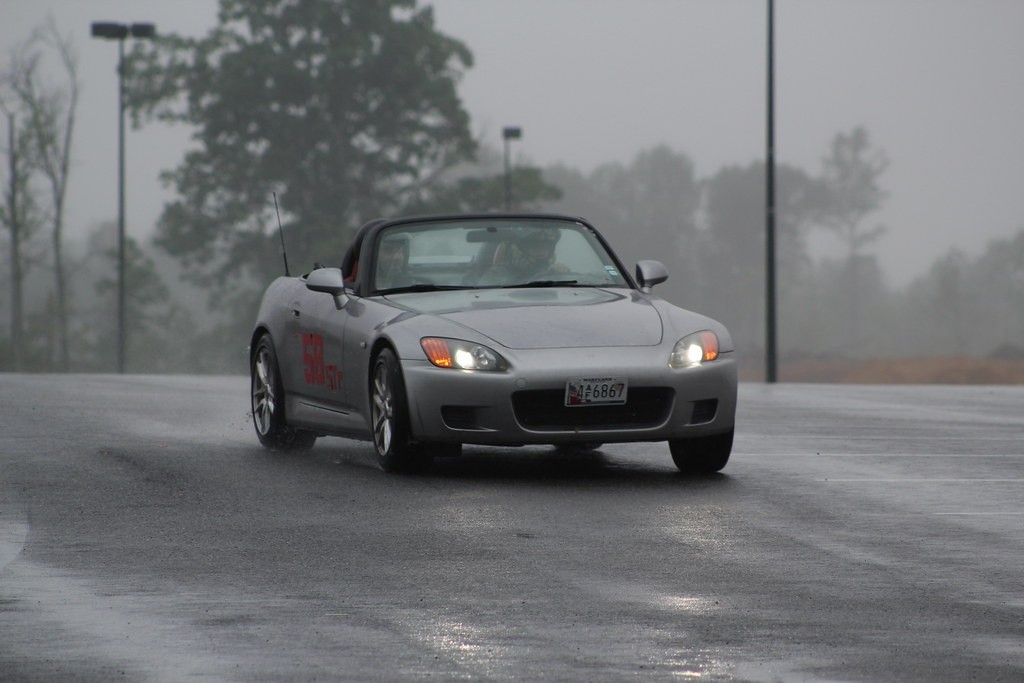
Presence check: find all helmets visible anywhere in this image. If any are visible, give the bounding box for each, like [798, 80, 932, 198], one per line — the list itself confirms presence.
[376, 233, 410, 277]
[504, 225, 561, 277]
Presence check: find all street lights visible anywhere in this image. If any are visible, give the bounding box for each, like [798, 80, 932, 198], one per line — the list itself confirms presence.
[87, 21, 157, 375]
[501, 126, 523, 214]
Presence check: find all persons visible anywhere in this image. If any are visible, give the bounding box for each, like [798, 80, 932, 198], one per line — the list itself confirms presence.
[478, 228, 571, 286]
[344, 219, 422, 290]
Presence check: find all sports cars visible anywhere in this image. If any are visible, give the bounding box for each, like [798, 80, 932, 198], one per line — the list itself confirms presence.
[246, 212, 740, 478]
